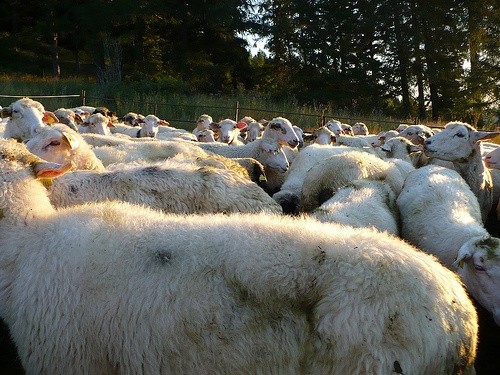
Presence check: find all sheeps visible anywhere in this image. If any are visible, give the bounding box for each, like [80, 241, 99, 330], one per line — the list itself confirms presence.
[0, 97, 305, 216]
[0, 134, 479, 375]
[272, 117, 500, 325]
